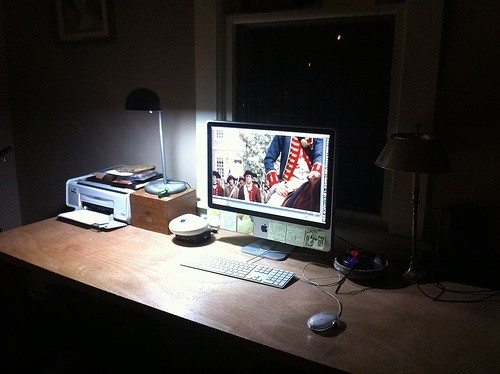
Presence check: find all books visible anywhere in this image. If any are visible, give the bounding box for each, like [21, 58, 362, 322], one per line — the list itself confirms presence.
[88, 164, 163, 190]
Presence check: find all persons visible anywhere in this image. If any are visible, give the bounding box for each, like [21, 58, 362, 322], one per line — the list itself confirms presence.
[263, 135, 323, 206]
[212, 170, 266, 203]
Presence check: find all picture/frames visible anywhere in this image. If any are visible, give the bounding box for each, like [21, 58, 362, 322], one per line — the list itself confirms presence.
[52, 0, 117, 47]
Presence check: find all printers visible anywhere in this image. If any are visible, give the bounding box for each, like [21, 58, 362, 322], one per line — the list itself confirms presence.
[58, 165, 165, 230]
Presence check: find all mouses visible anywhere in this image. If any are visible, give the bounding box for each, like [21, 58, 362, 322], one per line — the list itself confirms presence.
[306, 310, 340, 332]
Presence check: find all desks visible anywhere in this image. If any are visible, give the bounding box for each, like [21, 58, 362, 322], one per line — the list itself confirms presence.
[0, 213, 500, 374]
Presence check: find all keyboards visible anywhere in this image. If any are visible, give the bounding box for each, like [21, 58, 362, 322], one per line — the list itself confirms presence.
[180, 251, 295, 289]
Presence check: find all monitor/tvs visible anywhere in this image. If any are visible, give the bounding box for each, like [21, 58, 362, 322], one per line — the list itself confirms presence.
[207, 121, 336, 261]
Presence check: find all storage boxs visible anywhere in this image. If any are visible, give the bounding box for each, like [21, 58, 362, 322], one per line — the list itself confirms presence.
[130, 186, 197, 235]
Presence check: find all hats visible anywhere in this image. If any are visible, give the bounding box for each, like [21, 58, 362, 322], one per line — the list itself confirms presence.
[243, 170, 258, 177]
[239, 176, 244, 182]
[213, 171, 221, 178]
[226, 175, 238, 182]
[252, 177, 260, 188]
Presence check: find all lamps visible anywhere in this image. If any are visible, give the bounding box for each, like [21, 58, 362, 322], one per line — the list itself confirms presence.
[125, 89, 187, 194]
[374, 124, 455, 278]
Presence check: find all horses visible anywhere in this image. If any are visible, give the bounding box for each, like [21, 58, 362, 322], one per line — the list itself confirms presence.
[277, 174, 320, 213]
